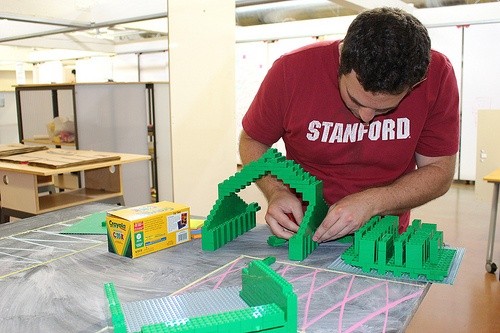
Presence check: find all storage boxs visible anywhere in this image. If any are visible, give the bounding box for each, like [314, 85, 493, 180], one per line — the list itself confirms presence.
[106, 201, 191, 259]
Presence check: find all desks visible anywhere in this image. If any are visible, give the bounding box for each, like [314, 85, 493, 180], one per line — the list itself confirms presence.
[482, 168, 500, 273]
[0, 145, 151, 224]
[0, 203, 432, 333]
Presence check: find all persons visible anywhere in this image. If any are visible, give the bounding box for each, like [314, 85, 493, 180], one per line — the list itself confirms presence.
[239, 7, 461, 243]
